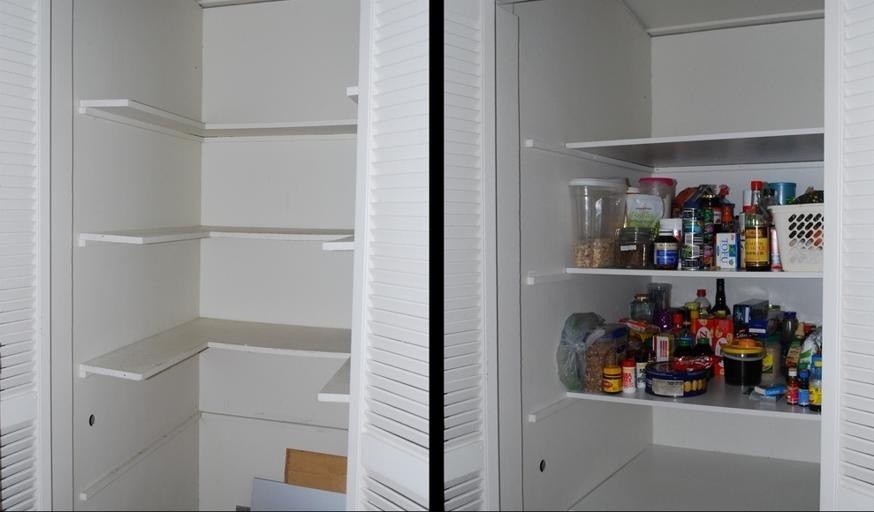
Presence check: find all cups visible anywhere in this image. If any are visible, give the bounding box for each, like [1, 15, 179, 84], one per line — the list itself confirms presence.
[648, 281, 671, 313]
[681, 202, 705, 271]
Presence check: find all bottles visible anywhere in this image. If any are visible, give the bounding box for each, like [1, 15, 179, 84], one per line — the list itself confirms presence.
[614, 227, 652, 268]
[713, 206, 739, 271]
[630, 294, 654, 322]
[671, 338, 713, 379]
[744, 179, 769, 270]
[786, 361, 822, 413]
[653, 229, 679, 269]
[602, 366, 621, 394]
[622, 352, 649, 394]
[782, 311, 799, 370]
[710, 277, 730, 319]
[694, 289, 710, 317]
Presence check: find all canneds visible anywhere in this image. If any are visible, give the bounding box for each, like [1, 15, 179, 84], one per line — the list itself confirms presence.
[700, 193, 723, 233]
[604, 366, 621, 393]
